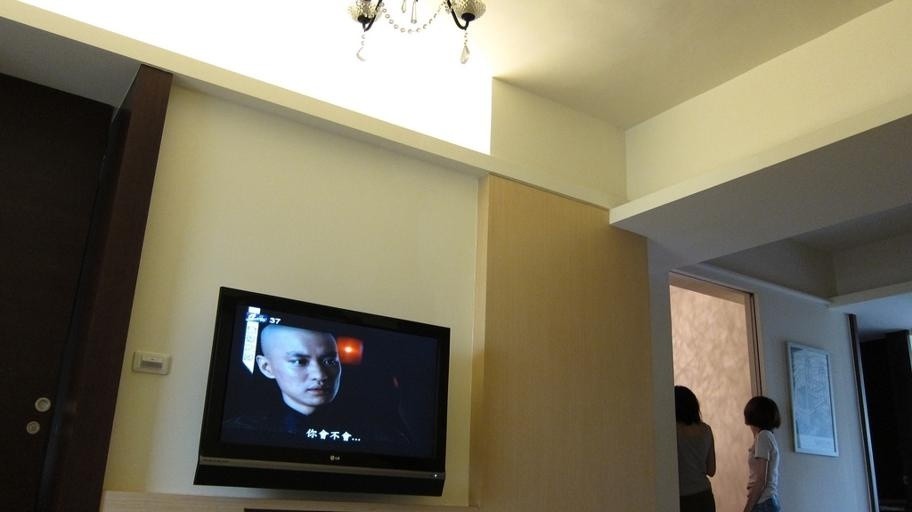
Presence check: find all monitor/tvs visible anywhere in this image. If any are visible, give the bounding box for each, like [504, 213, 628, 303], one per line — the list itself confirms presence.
[193, 286, 451, 496]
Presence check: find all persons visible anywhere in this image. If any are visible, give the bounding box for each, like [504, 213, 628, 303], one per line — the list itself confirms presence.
[742, 395, 784, 512]
[251, 318, 359, 441]
[674, 386, 719, 512]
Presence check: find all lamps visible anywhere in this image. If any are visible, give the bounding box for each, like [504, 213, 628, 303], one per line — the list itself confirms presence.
[343, 0, 485, 67]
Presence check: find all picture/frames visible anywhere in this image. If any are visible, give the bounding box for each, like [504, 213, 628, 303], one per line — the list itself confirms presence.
[786, 340, 839, 458]
